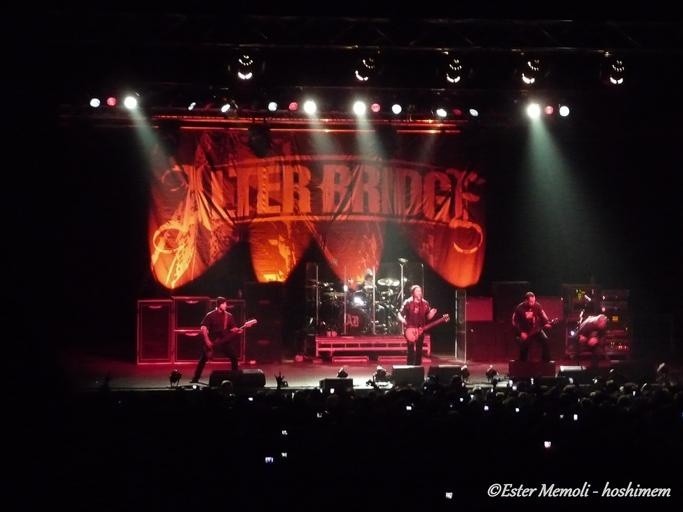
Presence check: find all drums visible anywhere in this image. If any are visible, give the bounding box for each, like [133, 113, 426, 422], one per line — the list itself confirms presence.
[305, 279, 323, 298]
[349, 291, 368, 309]
[338, 307, 371, 333]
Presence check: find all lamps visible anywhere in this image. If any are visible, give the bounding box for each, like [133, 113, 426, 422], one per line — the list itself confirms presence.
[555, 100, 577, 123]
[212, 94, 231, 113]
[434, 103, 449, 121]
[445, 56, 462, 84]
[287, 96, 299, 112]
[463, 101, 481, 121]
[237, 53, 255, 81]
[604, 48, 625, 86]
[388, 100, 406, 116]
[186, 98, 197, 111]
[354, 51, 380, 82]
[264, 95, 280, 112]
[522, 57, 540, 86]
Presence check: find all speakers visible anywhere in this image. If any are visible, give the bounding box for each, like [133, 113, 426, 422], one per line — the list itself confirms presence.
[391, 364, 424, 385]
[428, 364, 461, 385]
[319, 378, 354, 389]
[558, 366, 587, 378]
[209, 368, 266, 389]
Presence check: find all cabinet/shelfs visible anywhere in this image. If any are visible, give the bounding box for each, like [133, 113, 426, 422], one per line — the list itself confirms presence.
[560, 283, 606, 361]
[314, 335, 432, 358]
[598, 288, 634, 359]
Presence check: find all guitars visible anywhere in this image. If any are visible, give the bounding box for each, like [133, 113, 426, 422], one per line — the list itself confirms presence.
[520, 316, 560, 342]
[203, 319, 257, 359]
[404, 314, 450, 342]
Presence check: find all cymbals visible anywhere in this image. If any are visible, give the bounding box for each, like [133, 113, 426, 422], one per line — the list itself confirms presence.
[378, 278, 400, 287]
[324, 291, 345, 297]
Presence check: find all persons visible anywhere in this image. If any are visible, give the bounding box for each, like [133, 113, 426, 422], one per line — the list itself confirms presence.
[511, 292, 552, 362]
[20, 373, 681, 511]
[574, 313, 611, 365]
[399, 285, 438, 365]
[191, 297, 242, 382]
